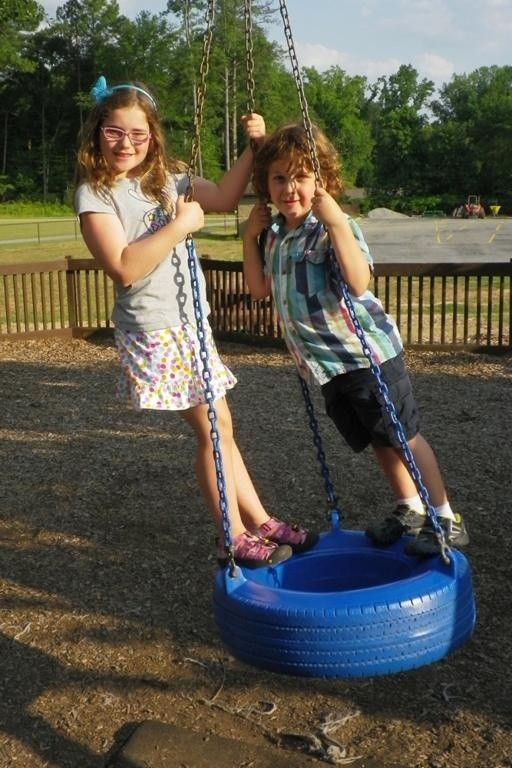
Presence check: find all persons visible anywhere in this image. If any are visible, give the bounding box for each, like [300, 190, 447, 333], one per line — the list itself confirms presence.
[74, 74, 319, 570]
[243, 124, 470, 559]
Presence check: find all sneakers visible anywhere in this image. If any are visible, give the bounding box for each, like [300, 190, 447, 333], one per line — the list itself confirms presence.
[254, 515, 320, 552]
[405, 512, 470, 556]
[365, 503, 430, 545]
[215, 530, 293, 569]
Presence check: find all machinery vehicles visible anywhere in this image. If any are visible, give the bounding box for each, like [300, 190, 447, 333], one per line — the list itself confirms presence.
[462, 195, 485, 219]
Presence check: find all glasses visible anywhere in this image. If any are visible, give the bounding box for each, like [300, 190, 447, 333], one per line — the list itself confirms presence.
[95, 116, 154, 145]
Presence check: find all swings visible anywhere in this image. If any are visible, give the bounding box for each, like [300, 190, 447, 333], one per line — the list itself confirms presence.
[182, 0, 478, 679]
